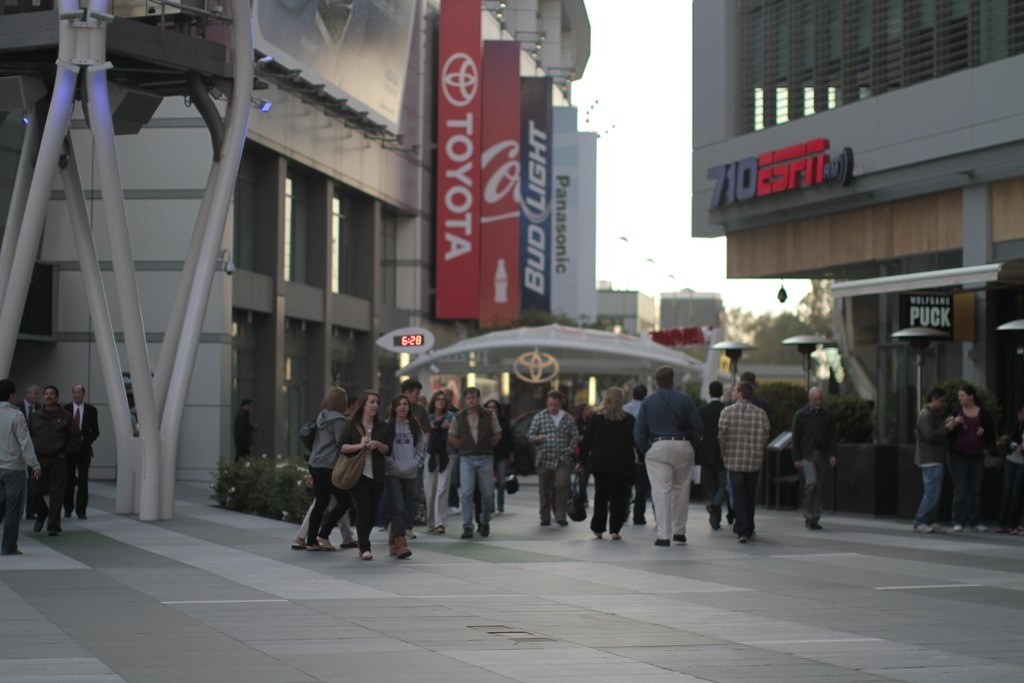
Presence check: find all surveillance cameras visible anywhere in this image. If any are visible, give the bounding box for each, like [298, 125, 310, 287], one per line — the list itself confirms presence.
[224, 260, 235, 275]
[778, 288, 787, 303]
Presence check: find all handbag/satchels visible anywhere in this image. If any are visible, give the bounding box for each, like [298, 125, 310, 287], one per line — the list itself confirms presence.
[331, 450, 366, 490]
[567, 493, 587, 521]
[300, 420, 316, 452]
[505, 478, 519, 494]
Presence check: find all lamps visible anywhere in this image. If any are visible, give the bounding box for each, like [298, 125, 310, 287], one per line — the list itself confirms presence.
[330, 112, 368, 122]
[364, 134, 405, 146]
[380, 144, 420, 155]
[278, 84, 325, 97]
[257, 67, 302, 80]
[303, 98, 347, 111]
[347, 124, 387, 135]
[481, 0, 577, 92]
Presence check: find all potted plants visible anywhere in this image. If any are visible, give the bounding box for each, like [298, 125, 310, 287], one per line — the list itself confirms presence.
[758, 374, 1007, 521]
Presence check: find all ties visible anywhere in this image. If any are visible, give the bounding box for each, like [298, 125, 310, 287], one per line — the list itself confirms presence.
[74, 407, 80, 428]
[28, 406, 33, 417]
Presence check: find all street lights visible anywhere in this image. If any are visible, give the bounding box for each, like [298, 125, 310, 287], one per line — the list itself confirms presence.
[890, 326, 949, 415]
[712, 341, 753, 389]
[781, 335, 832, 392]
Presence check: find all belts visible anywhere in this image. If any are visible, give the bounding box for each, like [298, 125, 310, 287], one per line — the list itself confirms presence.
[652, 436, 690, 442]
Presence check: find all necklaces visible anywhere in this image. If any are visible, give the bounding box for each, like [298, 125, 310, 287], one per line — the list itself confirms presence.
[362, 423, 372, 432]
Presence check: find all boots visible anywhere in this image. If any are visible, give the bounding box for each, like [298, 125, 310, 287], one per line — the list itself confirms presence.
[393, 536, 412, 558]
[388, 533, 396, 555]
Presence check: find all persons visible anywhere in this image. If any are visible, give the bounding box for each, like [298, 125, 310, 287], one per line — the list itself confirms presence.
[913, 388, 953, 534]
[130, 414, 140, 438]
[234, 399, 257, 462]
[339, 389, 393, 560]
[717, 382, 769, 542]
[28, 385, 81, 535]
[19, 385, 40, 520]
[291, 369, 840, 561]
[448, 388, 502, 538]
[303, 387, 348, 551]
[386, 393, 426, 557]
[576, 386, 638, 539]
[63, 384, 99, 520]
[424, 391, 454, 534]
[633, 367, 703, 546]
[792, 387, 837, 530]
[526, 390, 578, 526]
[0, 379, 40, 555]
[949, 383, 1024, 535]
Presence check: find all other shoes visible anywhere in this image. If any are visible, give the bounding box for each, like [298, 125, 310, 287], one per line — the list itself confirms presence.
[806, 519, 823, 530]
[673, 534, 687, 542]
[428, 527, 446, 533]
[291, 537, 373, 560]
[913, 520, 1024, 533]
[541, 520, 550, 525]
[612, 533, 621, 540]
[634, 518, 646, 524]
[655, 539, 669, 546]
[592, 530, 602, 538]
[0, 509, 88, 555]
[557, 520, 568, 525]
[461, 528, 474, 538]
[480, 525, 490, 536]
[406, 529, 416, 539]
[706, 504, 757, 543]
